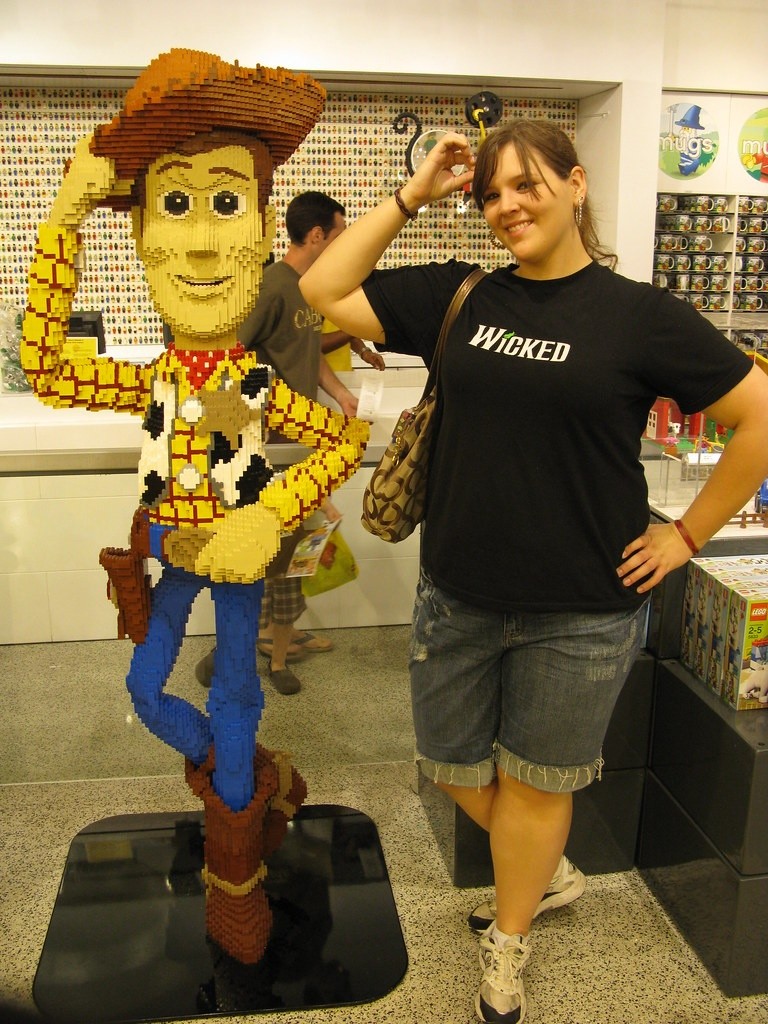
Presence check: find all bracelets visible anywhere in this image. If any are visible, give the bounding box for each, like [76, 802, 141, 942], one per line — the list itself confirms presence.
[674, 519, 700, 556]
[394, 186, 419, 221]
[358, 347, 372, 359]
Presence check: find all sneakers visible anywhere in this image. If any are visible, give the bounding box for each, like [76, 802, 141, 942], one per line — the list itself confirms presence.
[467, 855, 586, 933]
[475, 921, 527, 1024]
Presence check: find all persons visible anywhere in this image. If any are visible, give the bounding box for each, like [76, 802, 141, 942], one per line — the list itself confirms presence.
[235, 190, 356, 660]
[17, 46, 370, 967]
[196, 492, 342, 697]
[318, 318, 386, 371]
[295, 115, 768, 1024]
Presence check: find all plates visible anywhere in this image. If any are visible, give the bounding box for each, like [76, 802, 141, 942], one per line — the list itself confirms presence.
[410, 130, 465, 175]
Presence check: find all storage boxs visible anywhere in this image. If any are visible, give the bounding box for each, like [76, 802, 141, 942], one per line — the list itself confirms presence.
[678, 553, 768, 710]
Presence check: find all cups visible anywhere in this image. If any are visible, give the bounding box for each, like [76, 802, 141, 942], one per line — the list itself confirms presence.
[737, 217, 768, 234]
[733, 275, 768, 292]
[656, 254, 728, 270]
[735, 237, 768, 252]
[659, 234, 713, 252]
[653, 274, 729, 291]
[658, 195, 728, 212]
[660, 214, 730, 232]
[736, 256, 764, 272]
[739, 197, 768, 213]
[723, 332, 768, 351]
[733, 294, 763, 310]
[671, 293, 726, 309]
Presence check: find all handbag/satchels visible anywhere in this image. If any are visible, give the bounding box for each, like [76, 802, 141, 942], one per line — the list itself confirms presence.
[360, 268, 488, 543]
[301, 531, 360, 597]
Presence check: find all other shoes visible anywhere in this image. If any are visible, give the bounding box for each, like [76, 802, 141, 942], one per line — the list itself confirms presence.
[196, 647, 215, 687]
[265, 660, 300, 694]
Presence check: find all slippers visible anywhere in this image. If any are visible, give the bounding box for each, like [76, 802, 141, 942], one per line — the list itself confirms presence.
[294, 633, 333, 652]
[256, 638, 305, 662]
